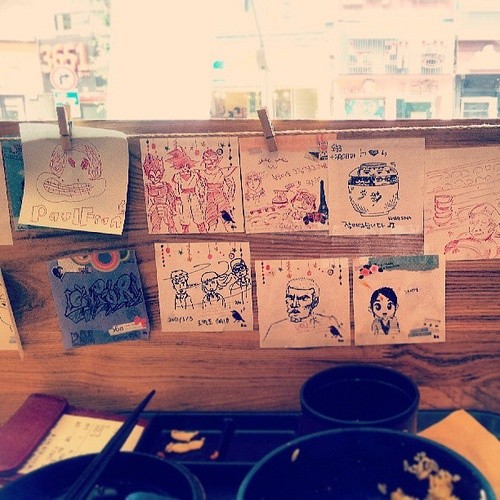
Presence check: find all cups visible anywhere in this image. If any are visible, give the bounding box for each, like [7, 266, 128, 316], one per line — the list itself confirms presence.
[300, 362, 420, 436]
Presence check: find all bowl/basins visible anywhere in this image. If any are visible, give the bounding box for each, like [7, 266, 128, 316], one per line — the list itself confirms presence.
[0, 451, 207, 500]
[237, 427, 497, 500]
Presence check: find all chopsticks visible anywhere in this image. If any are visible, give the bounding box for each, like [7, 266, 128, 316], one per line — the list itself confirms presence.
[59, 388, 157, 500]
